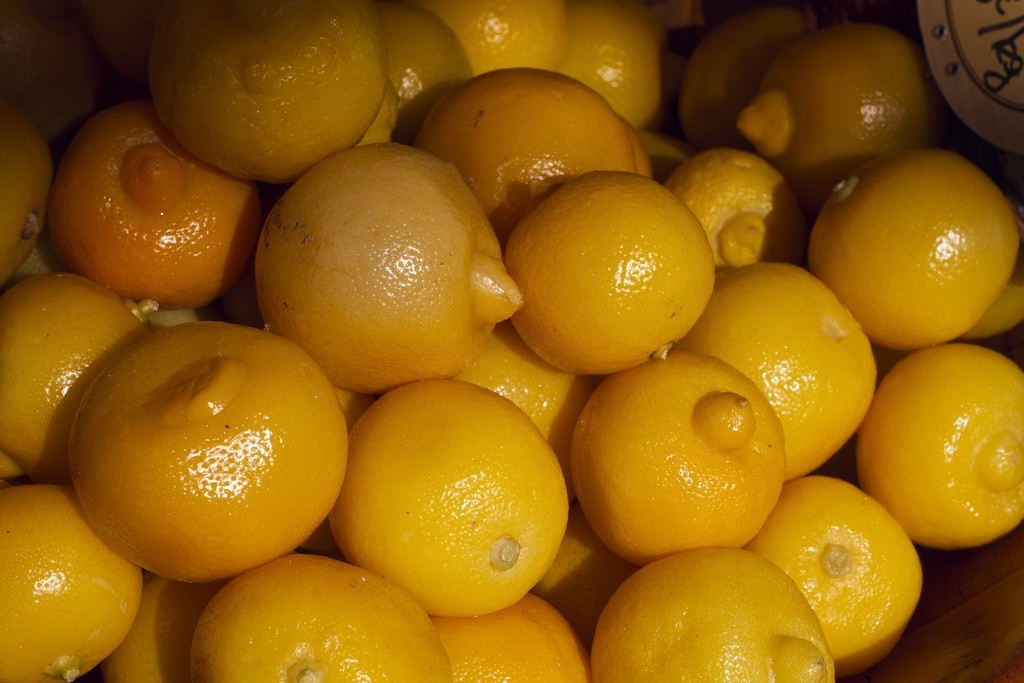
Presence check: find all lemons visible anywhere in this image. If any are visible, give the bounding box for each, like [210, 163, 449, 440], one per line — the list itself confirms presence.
[0, 0, 1024, 683]
